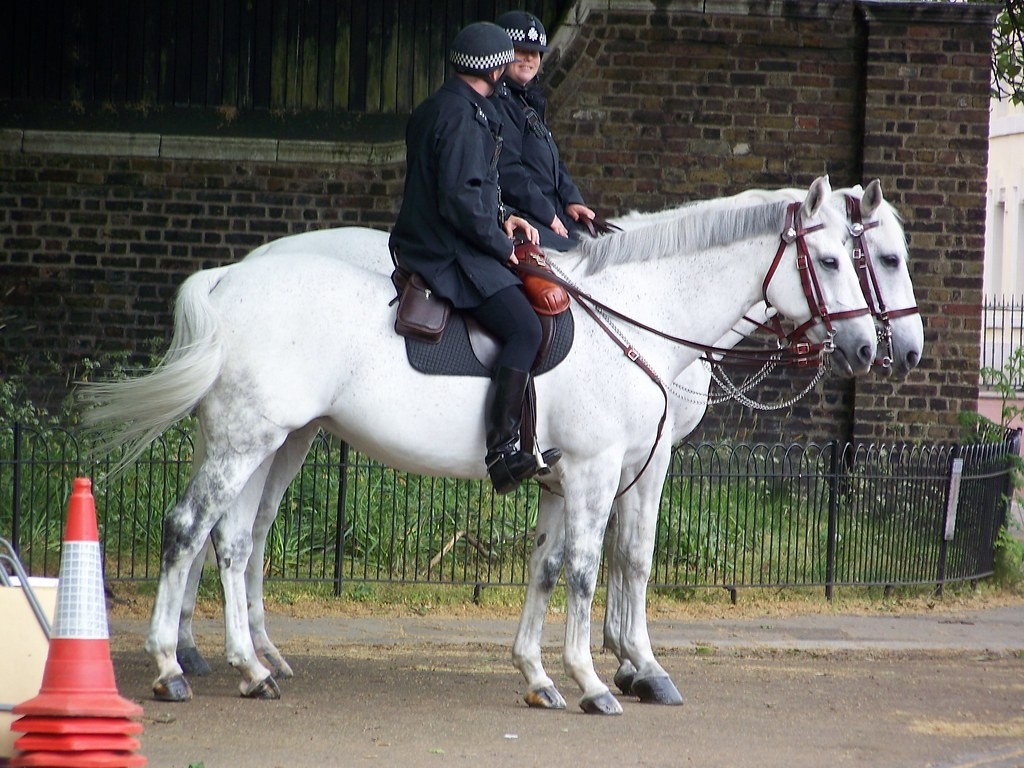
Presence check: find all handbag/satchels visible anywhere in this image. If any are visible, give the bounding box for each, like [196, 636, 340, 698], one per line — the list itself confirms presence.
[393, 272, 452, 345]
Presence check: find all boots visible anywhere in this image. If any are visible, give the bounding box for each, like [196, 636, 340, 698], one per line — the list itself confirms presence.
[485, 365, 563, 494]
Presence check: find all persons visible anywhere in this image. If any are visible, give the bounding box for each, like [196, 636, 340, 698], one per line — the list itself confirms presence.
[392, 22, 562, 494]
[486, 11, 596, 253]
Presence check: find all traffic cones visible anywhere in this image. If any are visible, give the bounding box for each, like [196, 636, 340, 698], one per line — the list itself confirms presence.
[10, 476, 146, 767]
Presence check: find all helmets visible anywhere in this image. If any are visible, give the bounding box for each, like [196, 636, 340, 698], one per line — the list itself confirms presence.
[495, 10, 550, 52]
[447, 20, 515, 72]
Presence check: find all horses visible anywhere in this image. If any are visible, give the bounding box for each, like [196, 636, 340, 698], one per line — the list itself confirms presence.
[77, 170, 925, 714]
